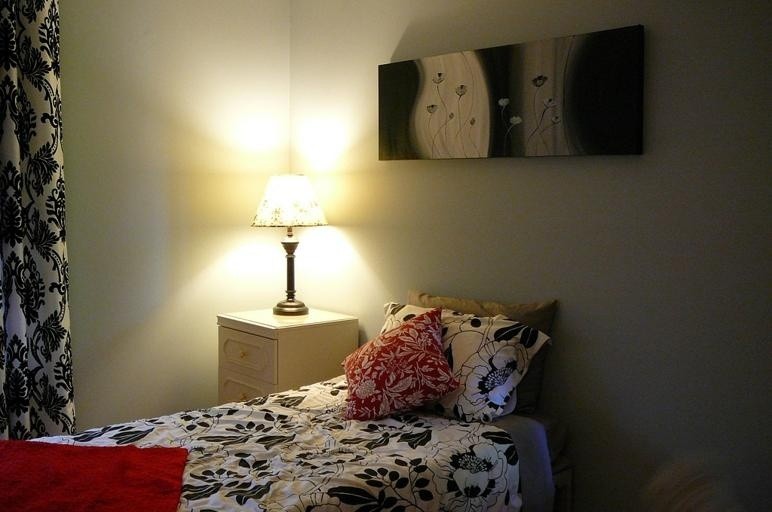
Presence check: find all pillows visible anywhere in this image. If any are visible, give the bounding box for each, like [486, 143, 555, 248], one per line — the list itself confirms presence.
[336, 305, 462, 426]
[377, 300, 553, 426]
[406, 292, 557, 412]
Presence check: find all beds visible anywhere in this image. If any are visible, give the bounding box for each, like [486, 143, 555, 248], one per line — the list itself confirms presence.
[1, 291, 560, 512]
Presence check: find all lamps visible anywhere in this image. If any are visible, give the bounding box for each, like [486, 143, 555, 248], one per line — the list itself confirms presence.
[251, 172, 332, 318]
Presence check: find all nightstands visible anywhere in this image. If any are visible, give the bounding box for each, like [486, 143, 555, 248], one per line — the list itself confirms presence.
[215, 310, 360, 411]
